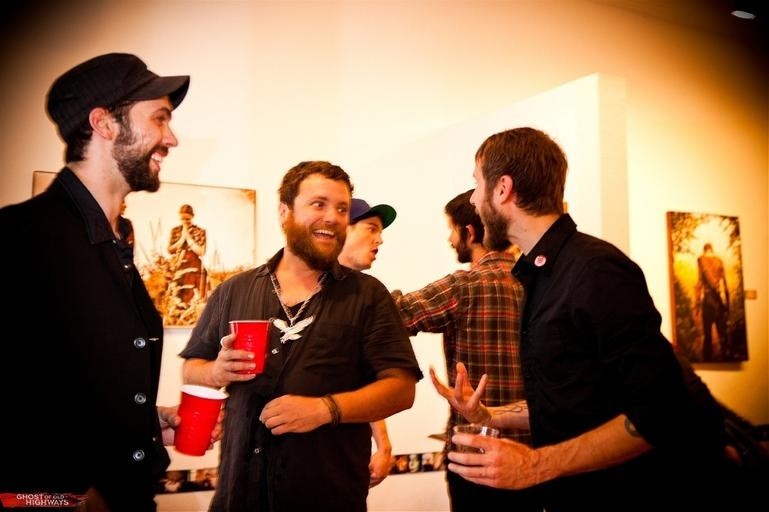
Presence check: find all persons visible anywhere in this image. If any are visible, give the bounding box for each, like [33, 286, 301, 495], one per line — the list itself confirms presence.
[693, 242, 732, 364]
[338, 198, 397, 490]
[178, 161, 424, 511]
[391, 191, 522, 512]
[428, 127, 728, 510]
[169, 204, 206, 305]
[119, 200, 135, 270]
[2, 54, 191, 511]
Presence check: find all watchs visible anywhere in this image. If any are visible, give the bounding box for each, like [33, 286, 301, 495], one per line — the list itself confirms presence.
[324, 395, 342, 428]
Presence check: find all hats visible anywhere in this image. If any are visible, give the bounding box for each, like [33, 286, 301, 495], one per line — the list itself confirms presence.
[45, 51, 191, 128]
[350, 198, 397, 230]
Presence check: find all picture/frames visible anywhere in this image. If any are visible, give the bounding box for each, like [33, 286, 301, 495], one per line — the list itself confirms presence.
[32, 170, 256, 329]
[666, 210, 749, 368]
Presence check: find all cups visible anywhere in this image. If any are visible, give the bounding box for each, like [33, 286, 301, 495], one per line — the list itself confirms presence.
[173, 383, 229, 458]
[228, 320, 273, 374]
[453, 424, 500, 455]
